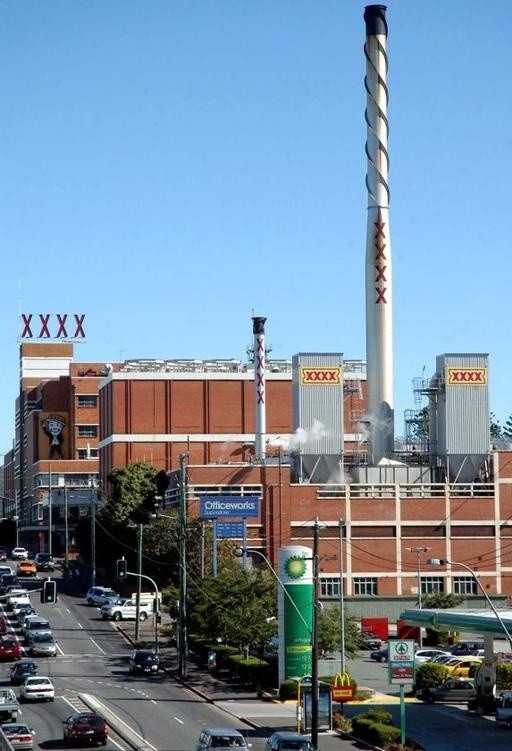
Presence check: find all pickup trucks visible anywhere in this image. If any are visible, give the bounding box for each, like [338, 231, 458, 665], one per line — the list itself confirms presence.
[0, 686, 20, 721]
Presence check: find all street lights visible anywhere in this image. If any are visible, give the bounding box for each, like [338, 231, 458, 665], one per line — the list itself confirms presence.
[405, 545, 434, 610]
[68, 493, 96, 585]
[337, 516, 352, 675]
[424, 555, 512, 649]
[150, 513, 186, 675]
[234, 548, 319, 750]
[0, 515, 20, 523]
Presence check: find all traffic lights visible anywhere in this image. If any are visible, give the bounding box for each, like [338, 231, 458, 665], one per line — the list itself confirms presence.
[44, 581, 55, 603]
[117, 559, 127, 580]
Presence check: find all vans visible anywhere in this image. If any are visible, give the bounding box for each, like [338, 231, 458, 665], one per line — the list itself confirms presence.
[100, 597, 154, 623]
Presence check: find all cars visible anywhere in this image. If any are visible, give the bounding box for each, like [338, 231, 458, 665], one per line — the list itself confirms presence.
[19, 674, 57, 703]
[11, 547, 28, 562]
[364, 632, 512, 730]
[128, 649, 161, 679]
[17, 559, 36, 578]
[0, 565, 58, 660]
[1, 722, 35, 751]
[9, 660, 40, 686]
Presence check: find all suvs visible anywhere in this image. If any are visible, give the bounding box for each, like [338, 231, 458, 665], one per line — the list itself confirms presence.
[195, 725, 252, 750]
[0, 549, 7, 561]
[86, 585, 120, 608]
[35, 551, 55, 573]
[59, 711, 111, 745]
[264, 731, 314, 751]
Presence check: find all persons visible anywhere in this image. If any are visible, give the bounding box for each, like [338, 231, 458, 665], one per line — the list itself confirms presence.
[41, 414, 67, 460]
[229, 739, 240, 747]
[298, 742, 313, 751]
